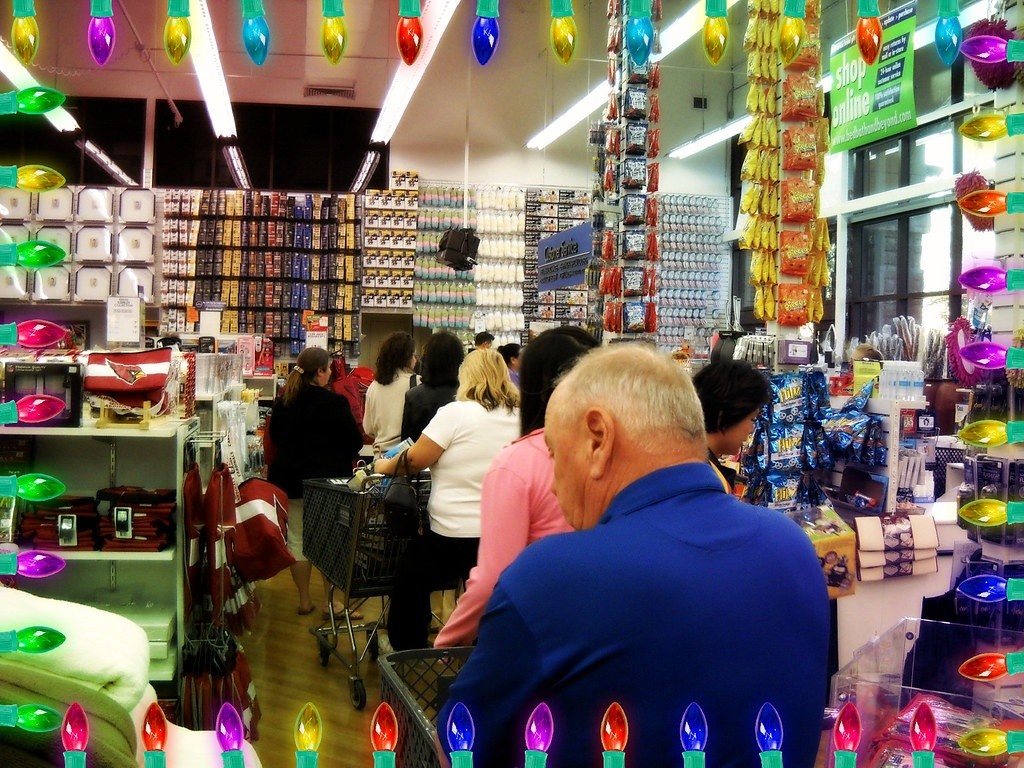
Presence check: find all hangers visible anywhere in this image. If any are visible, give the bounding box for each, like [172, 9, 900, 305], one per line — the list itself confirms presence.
[216, 440, 223, 472]
[187, 438, 197, 470]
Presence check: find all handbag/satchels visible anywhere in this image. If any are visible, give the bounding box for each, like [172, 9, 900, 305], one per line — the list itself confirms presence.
[382, 448, 425, 544]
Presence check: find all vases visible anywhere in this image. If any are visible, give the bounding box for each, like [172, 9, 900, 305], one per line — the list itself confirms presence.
[934, 379, 964, 435]
[924, 378, 942, 416]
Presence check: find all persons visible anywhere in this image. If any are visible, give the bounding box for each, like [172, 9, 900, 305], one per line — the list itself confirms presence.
[373, 348, 520, 593]
[362, 333, 424, 456]
[543, 292, 554, 302]
[848, 344, 884, 369]
[433, 327, 601, 667]
[575, 293, 586, 303]
[542, 307, 553, 318]
[266, 348, 364, 621]
[401, 331, 467, 443]
[692, 357, 774, 497]
[466, 331, 496, 354]
[430, 341, 828, 768]
[496, 343, 522, 388]
[575, 308, 585, 318]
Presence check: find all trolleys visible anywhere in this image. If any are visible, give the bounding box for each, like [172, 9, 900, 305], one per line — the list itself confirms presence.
[302, 472, 482, 710]
[376, 647, 476, 768]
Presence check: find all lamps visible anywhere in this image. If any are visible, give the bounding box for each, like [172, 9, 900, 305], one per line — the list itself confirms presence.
[348, 149, 381, 193]
[222, 145, 253, 189]
[662, 0, 1007, 159]
[0, 41, 81, 133]
[526, 1, 739, 151]
[366, 0, 460, 145]
[185, 1, 238, 140]
[76, 138, 140, 186]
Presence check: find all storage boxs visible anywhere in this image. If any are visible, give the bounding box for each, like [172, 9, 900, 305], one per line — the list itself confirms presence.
[898, 453, 926, 489]
[4, 362, 82, 428]
[81, 598, 177, 641]
[149, 641, 171, 660]
[825, 615, 1024, 768]
[837, 466, 889, 514]
[777, 339, 811, 364]
[326, 437, 415, 492]
[853, 359, 881, 398]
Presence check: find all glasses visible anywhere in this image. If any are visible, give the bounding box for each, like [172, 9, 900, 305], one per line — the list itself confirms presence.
[411, 353, 420, 360]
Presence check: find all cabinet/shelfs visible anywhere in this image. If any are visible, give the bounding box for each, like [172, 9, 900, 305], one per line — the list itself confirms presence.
[0, 358, 277, 682]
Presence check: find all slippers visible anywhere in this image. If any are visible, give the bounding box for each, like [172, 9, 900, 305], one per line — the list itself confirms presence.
[297, 602, 315, 615]
[322, 605, 364, 620]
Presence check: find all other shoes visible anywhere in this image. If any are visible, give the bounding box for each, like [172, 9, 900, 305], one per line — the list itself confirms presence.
[376, 628, 398, 666]
